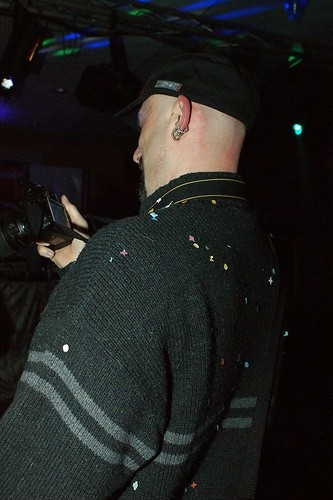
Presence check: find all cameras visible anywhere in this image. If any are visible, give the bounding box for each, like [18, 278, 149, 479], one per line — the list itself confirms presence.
[0, 178, 75, 257]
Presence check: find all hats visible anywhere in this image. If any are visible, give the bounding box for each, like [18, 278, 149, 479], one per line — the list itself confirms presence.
[114, 52, 259, 131]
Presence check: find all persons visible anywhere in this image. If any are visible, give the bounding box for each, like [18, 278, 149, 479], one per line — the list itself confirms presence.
[0, 50, 286, 500]
